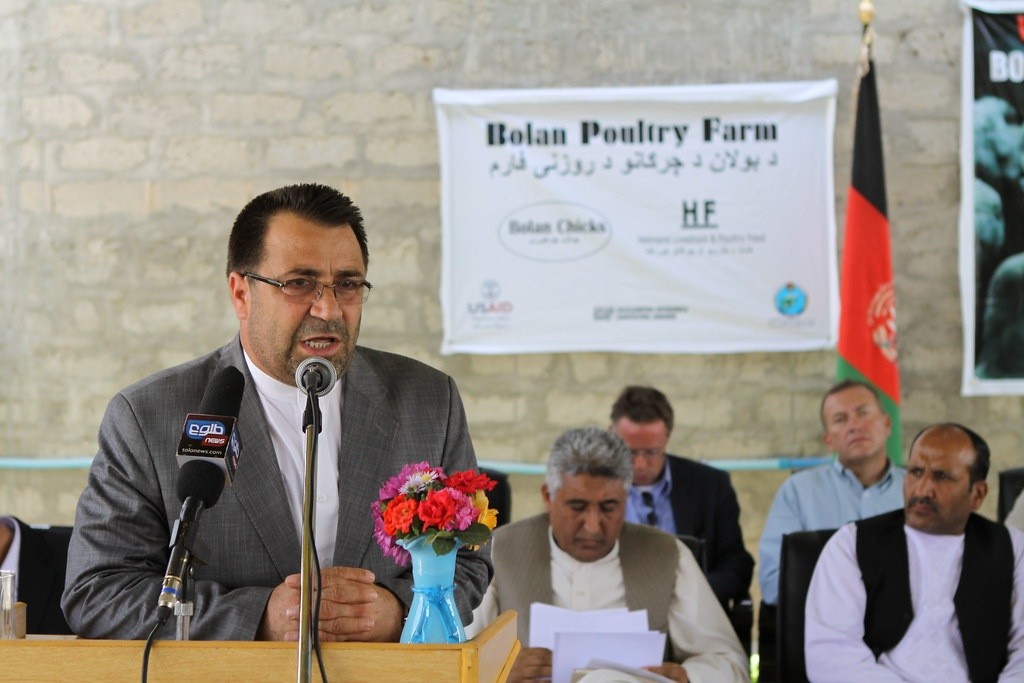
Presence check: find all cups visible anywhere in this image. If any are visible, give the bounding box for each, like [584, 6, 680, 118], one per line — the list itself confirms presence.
[0, 570, 16, 639]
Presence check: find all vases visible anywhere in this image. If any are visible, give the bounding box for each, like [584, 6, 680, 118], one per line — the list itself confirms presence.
[393, 534, 465, 643]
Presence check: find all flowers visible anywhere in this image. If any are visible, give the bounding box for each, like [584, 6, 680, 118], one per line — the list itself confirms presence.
[368, 467, 489, 565]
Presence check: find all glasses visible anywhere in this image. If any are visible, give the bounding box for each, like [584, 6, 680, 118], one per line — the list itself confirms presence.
[240, 271, 373, 305]
[642, 492, 658, 525]
[628, 447, 664, 458]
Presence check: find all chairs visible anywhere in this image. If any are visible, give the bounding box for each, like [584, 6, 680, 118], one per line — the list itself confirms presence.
[481, 465, 1024, 682]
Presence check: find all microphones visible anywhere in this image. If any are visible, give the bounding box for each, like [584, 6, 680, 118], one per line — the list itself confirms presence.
[295, 356, 337, 396]
[155, 366, 246, 624]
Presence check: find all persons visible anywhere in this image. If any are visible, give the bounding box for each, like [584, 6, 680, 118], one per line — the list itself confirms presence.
[60, 182, 492, 643]
[491, 426, 753, 683]
[753, 379, 909, 608]
[0, 514, 74, 634]
[607, 385, 755, 605]
[803, 423, 1024, 683]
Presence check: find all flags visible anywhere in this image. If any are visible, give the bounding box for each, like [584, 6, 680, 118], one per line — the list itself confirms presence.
[831, 20, 907, 463]
